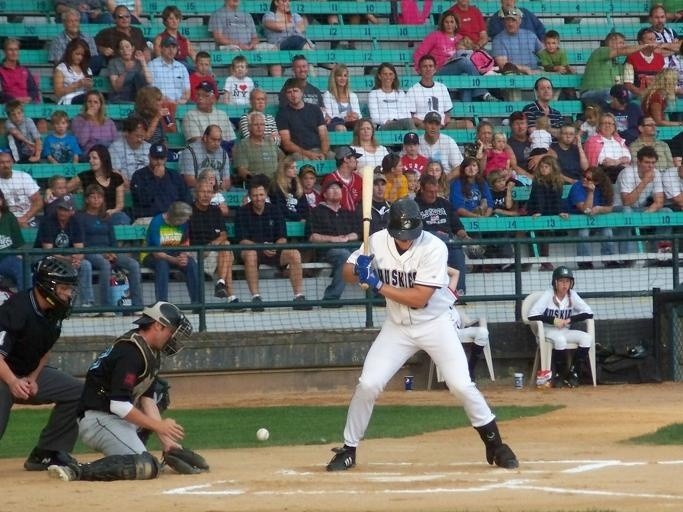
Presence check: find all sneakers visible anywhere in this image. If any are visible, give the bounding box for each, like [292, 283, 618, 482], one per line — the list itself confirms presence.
[326, 444, 356, 471]
[225, 298, 246, 312]
[292, 296, 312, 311]
[214, 281, 227, 299]
[555, 369, 579, 387]
[251, 296, 264, 311]
[320, 295, 343, 308]
[483, 437, 520, 469]
[22, 445, 84, 482]
[78, 302, 200, 316]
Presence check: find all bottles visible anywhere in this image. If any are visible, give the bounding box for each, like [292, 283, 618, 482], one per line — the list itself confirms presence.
[513, 372, 523, 389]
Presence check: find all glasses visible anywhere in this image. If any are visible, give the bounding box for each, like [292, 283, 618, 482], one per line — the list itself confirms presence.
[116, 12, 129, 18]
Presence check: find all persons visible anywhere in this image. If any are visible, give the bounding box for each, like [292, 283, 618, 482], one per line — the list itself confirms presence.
[552, 5, 682, 268]
[527, 266, 595, 390]
[323, 196, 520, 474]
[0, 255, 86, 471]
[45, 301, 194, 481]
[1, 1, 575, 314]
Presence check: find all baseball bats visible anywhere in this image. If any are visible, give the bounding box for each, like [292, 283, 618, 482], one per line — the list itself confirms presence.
[361, 166, 374, 289]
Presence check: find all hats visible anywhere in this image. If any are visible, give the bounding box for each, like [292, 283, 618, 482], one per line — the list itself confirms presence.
[158, 35, 177, 48]
[424, 112, 441, 124]
[55, 194, 74, 211]
[319, 180, 343, 194]
[403, 133, 418, 146]
[334, 145, 362, 160]
[609, 84, 630, 103]
[503, 8, 523, 19]
[149, 144, 167, 160]
[299, 164, 316, 175]
[373, 172, 387, 183]
[194, 80, 214, 93]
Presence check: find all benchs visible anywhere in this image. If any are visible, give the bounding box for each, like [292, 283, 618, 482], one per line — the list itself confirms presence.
[0, 0, 682, 310]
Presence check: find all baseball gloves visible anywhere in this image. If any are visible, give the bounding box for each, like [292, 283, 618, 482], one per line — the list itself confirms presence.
[163, 448, 209, 474]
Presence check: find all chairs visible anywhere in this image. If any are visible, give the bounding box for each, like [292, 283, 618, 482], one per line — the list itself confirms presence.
[424, 305, 496, 392]
[517, 289, 600, 388]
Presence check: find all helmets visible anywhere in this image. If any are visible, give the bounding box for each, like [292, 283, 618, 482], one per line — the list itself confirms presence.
[129, 299, 193, 358]
[387, 199, 423, 240]
[29, 255, 80, 321]
[551, 266, 574, 288]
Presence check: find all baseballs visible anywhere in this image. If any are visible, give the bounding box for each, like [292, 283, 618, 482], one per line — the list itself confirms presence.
[256, 428, 269, 440]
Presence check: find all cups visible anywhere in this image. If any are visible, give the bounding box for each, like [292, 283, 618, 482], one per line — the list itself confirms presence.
[403, 375, 414, 390]
[161, 111, 174, 128]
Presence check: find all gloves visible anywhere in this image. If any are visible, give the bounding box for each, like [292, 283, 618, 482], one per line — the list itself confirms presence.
[353, 254, 383, 293]
